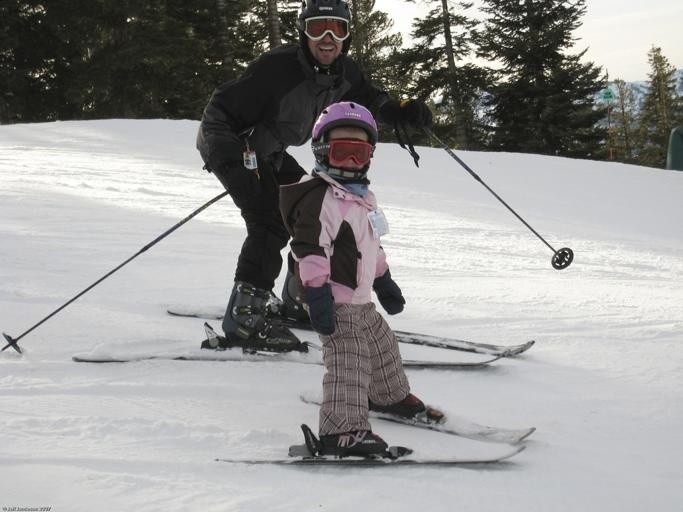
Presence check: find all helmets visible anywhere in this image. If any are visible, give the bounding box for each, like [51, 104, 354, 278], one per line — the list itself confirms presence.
[297, 0, 351, 54]
[311, 100, 379, 162]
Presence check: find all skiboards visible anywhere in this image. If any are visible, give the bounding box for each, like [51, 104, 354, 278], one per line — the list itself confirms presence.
[214, 395, 536, 465]
[73, 309, 534, 366]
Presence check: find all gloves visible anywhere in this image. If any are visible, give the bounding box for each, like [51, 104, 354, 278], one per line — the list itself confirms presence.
[372, 267, 405, 316]
[301, 281, 337, 336]
[379, 98, 433, 128]
[217, 158, 264, 207]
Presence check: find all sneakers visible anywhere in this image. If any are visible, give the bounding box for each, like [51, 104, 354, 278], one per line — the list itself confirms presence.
[368, 393, 427, 420]
[317, 429, 388, 454]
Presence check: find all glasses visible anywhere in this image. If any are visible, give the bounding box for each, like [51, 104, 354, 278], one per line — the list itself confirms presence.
[302, 15, 351, 42]
[326, 137, 372, 166]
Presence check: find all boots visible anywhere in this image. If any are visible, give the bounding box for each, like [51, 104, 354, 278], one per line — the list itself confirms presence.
[221, 278, 301, 347]
[281, 250, 311, 320]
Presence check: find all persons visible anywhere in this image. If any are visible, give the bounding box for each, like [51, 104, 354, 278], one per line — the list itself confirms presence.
[194, 0, 433, 351]
[277, 102, 426, 455]
